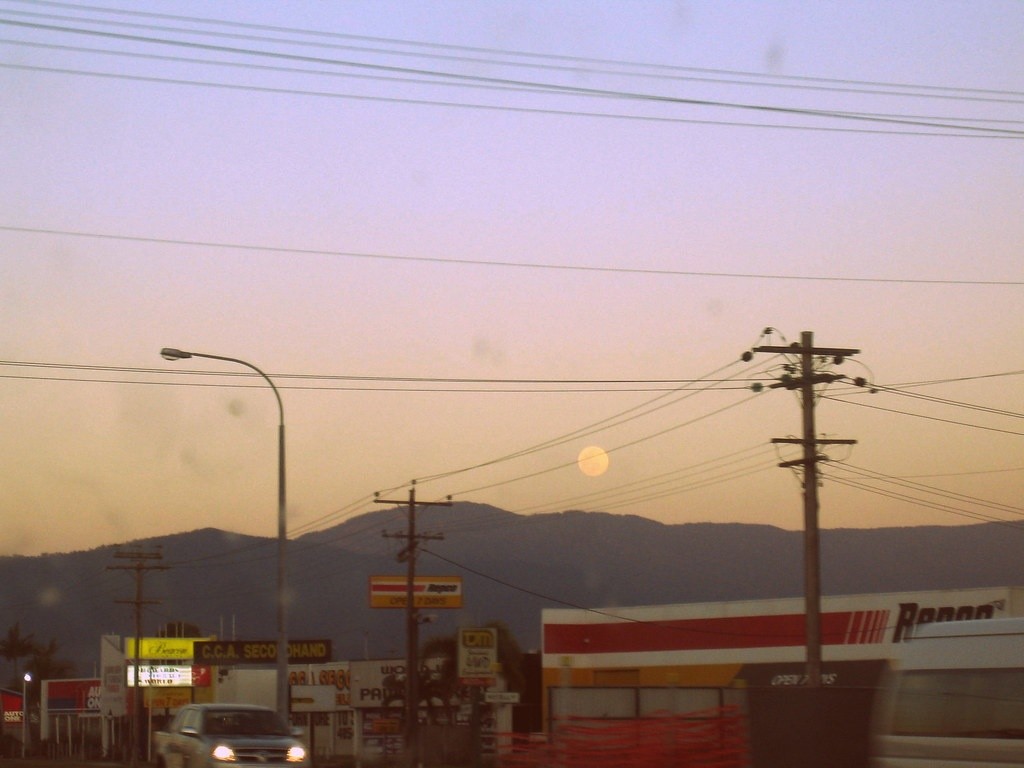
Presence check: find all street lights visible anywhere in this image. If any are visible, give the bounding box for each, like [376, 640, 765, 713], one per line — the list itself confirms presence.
[21, 670, 32, 761]
[160, 347, 289, 725]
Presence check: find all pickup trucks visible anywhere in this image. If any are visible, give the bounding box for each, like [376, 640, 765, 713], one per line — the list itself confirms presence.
[151, 703, 313, 768]
